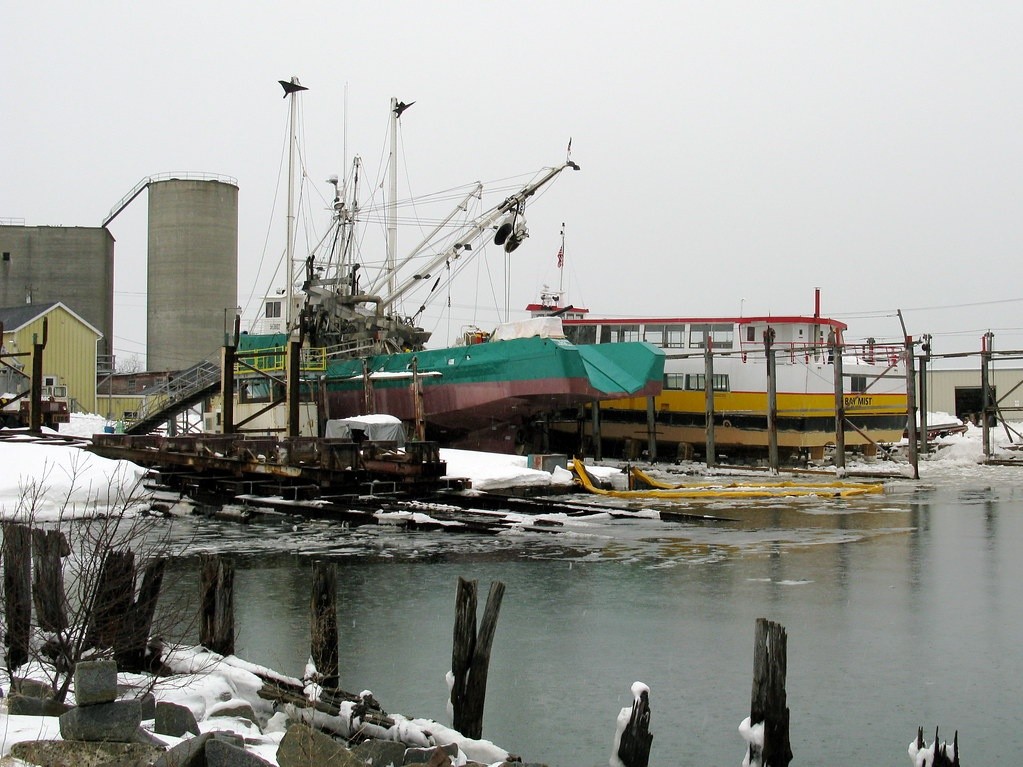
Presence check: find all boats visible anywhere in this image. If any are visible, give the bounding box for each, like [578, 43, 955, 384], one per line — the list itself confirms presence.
[456, 306, 908, 457]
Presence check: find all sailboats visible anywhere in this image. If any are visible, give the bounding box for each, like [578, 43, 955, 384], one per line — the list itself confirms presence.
[238, 72, 665, 456]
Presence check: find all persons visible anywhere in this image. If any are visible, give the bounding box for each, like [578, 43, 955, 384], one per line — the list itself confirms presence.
[400, 344, 410, 353]
